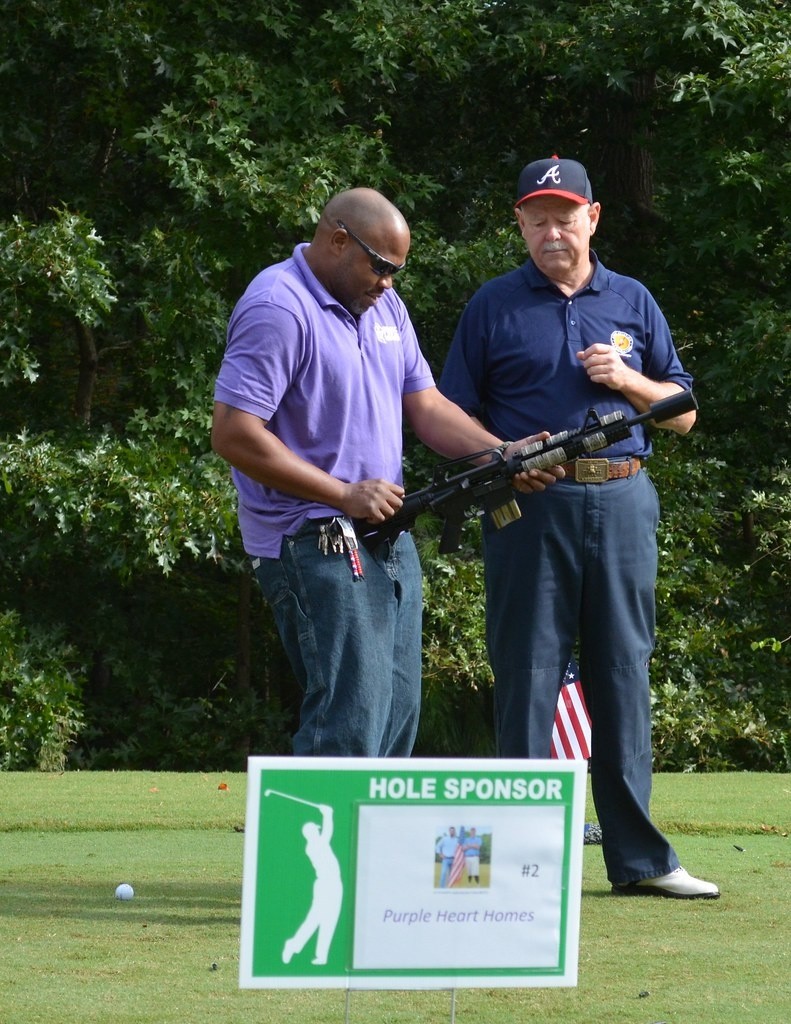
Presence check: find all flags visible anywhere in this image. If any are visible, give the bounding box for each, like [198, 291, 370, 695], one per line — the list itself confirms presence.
[548, 657, 593, 760]
[448, 827, 465, 887]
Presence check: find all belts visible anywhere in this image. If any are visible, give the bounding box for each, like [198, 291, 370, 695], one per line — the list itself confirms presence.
[562, 458, 642, 484]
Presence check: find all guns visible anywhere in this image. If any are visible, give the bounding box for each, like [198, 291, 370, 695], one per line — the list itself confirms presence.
[351, 389, 701, 555]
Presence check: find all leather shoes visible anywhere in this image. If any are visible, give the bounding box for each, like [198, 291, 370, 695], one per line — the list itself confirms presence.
[613, 865, 721, 900]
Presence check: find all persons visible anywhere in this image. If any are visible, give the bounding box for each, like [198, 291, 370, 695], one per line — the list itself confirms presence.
[435, 827, 459, 888]
[460, 828, 482, 883]
[440, 153, 722, 899]
[212, 187, 565, 756]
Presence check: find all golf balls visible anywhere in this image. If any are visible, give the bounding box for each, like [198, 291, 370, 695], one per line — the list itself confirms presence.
[114, 884, 134, 900]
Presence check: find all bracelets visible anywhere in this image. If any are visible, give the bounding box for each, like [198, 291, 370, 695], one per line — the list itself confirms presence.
[491, 442, 514, 459]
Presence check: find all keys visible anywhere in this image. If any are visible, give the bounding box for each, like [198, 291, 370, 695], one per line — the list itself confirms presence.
[317, 514, 359, 555]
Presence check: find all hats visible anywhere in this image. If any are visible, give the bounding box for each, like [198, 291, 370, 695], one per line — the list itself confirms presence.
[513, 156, 593, 208]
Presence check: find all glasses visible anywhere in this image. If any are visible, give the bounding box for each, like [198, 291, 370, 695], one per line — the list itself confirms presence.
[336, 219, 407, 276]
